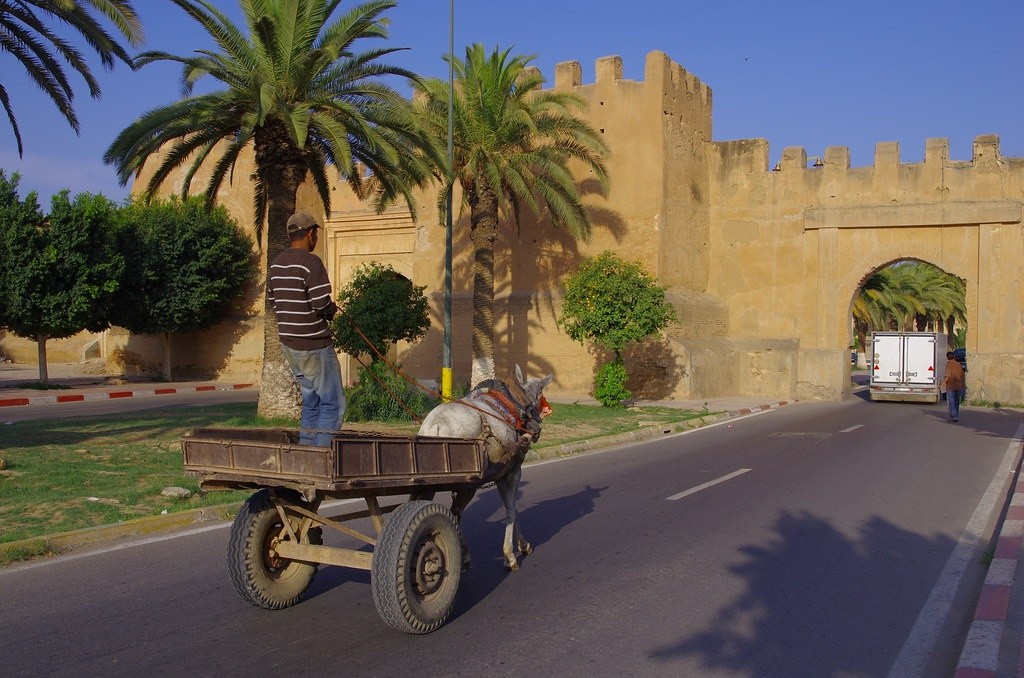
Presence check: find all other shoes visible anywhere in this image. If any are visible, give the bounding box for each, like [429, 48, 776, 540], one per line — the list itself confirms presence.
[952, 418, 958, 422]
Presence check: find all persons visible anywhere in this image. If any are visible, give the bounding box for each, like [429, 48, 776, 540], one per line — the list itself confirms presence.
[938, 352, 964, 421]
[267, 212, 345, 448]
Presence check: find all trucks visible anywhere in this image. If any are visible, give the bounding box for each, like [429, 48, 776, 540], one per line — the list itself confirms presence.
[870, 331, 947, 404]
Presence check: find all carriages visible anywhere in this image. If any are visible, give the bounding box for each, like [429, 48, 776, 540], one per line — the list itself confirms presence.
[180, 363, 554, 635]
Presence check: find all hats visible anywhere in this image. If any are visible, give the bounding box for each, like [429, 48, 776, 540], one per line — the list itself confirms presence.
[286, 213, 322, 234]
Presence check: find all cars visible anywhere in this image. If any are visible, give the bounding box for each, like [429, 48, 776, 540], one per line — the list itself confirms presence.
[954, 348, 966, 370]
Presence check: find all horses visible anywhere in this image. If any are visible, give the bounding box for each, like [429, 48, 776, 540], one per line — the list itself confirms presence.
[418, 363, 552, 571]
